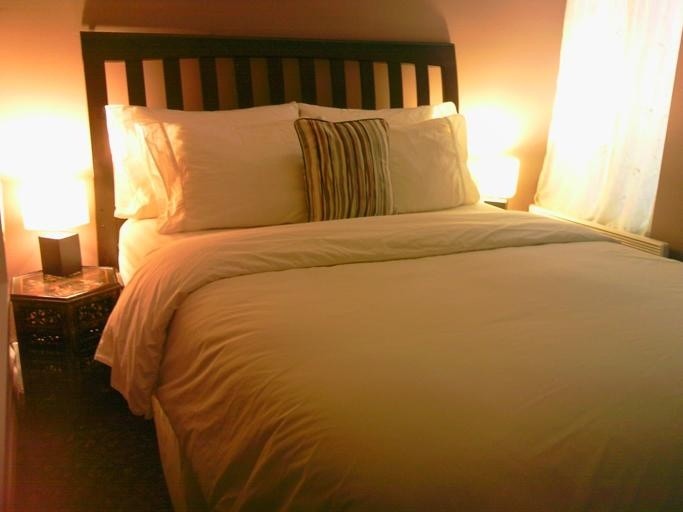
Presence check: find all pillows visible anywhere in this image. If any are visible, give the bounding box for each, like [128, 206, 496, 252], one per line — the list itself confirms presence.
[136, 122, 309, 233]
[390, 113, 480, 216]
[301, 101, 458, 128]
[101, 102, 300, 221]
[294, 118, 394, 223]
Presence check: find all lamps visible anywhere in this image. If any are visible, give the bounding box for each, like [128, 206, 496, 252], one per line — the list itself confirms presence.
[18, 177, 90, 277]
[468, 151, 518, 210]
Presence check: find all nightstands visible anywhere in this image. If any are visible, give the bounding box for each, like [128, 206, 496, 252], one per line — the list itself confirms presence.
[10, 267, 126, 413]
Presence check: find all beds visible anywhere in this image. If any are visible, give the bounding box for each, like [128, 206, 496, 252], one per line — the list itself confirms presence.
[79, 33, 683, 512]
